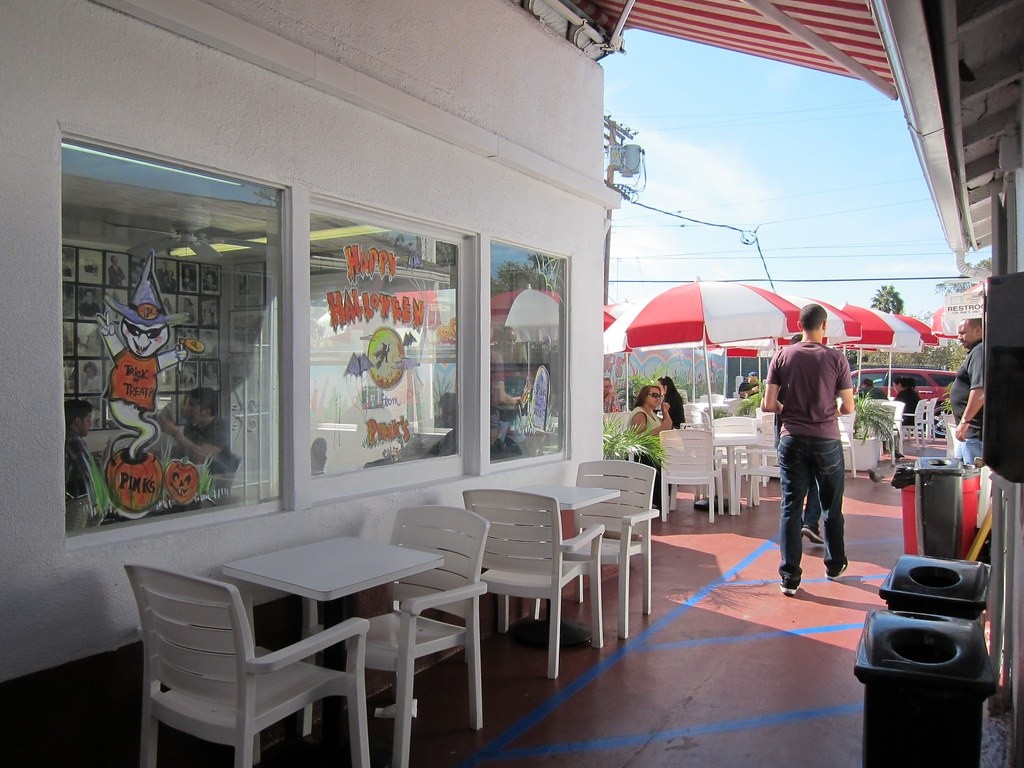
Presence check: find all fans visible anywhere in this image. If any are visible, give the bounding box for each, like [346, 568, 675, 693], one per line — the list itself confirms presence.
[100, 203, 270, 262]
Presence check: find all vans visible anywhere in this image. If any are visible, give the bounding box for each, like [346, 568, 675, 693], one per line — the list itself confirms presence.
[851, 368, 960, 437]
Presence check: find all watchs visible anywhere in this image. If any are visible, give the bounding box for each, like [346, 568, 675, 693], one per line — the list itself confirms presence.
[960, 418, 970, 424]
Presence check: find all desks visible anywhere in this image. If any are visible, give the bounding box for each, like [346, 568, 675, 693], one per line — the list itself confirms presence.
[514, 485, 621, 653]
[221, 536, 446, 768]
[714, 433, 757, 517]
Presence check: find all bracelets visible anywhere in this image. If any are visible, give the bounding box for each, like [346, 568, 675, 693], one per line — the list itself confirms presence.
[172, 429, 180, 437]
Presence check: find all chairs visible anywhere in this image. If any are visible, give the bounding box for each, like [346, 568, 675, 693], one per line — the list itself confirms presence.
[660, 396, 960, 524]
[562, 459, 659, 642]
[341, 503, 490, 768]
[122, 560, 372, 768]
[461, 488, 609, 681]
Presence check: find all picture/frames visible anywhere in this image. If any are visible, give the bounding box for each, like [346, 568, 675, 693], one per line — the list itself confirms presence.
[56, 243, 222, 432]
[228, 310, 270, 353]
[234, 263, 267, 306]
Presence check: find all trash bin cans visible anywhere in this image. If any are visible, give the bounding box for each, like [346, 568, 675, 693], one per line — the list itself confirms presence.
[854, 608, 996, 767]
[895, 463, 980, 559]
[878, 554, 992, 620]
[914, 457, 965, 558]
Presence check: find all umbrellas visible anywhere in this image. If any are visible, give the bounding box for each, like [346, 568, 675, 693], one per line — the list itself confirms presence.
[604, 277, 988, 424]
[490, 284, 562, 326]
[314, 289, 441, 344]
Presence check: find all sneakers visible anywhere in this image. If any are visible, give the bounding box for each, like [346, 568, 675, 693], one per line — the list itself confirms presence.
[825, 555, 848, 580]
[780, 580, 799, 595]
[801, 524, 824, 544]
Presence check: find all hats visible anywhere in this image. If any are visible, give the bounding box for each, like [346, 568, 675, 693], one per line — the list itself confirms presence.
[749, 372, 758, 376]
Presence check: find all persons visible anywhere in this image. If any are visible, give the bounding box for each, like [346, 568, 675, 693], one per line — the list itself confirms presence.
[65, 399, 101, 496]
[603, 378, 620, 413]
[892, 377, 925, 426]
[658, 377, 686, 429]
[490, 328, 529, 455]
[764, 303, 855, 595]
[62, 246, 221, 432]
[858, 379, 873, 398]
[158, 387, 230, 499]
[950, 317, 983, 465]
[774, 334, 824, 544]
[738, 372, 759, 399]
[627, 385, 672, 511]
[425, 391, 457, 457]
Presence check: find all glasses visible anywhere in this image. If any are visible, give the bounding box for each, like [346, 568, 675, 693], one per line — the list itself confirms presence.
[489, 421, 501, 429]
[648, 393, 663, 398]
